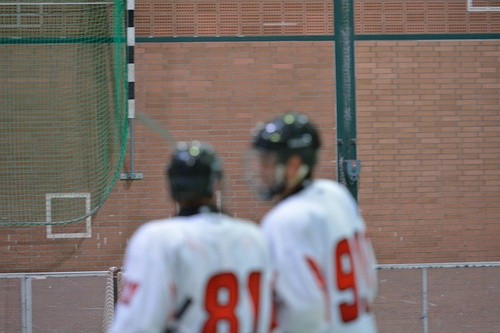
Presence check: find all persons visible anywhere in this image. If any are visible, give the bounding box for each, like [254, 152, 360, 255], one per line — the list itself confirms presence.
[245, 112, 380, 332]
[107, 138, 275, 332]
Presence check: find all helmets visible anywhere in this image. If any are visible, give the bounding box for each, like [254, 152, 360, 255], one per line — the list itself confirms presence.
[165, 137, 224, 197]
[252, 112, 322, 157]
